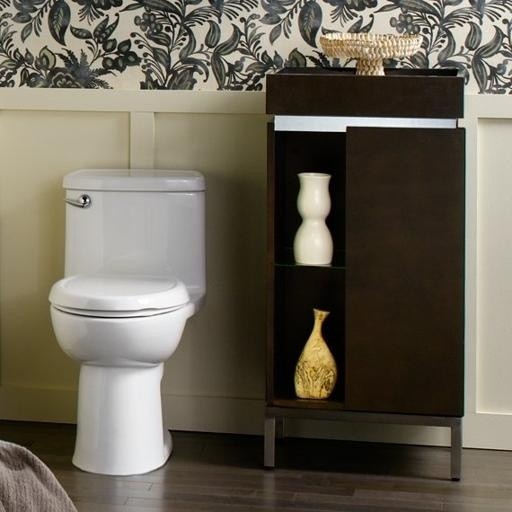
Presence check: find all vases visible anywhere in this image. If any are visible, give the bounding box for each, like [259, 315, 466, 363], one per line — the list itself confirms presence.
[293, 171, 338, 400]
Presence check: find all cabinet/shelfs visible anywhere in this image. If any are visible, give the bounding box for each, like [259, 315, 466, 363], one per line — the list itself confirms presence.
[265, 67, 465, 482]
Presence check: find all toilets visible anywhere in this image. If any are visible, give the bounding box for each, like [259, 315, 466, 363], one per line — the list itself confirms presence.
[46, 169, 207, 476]
